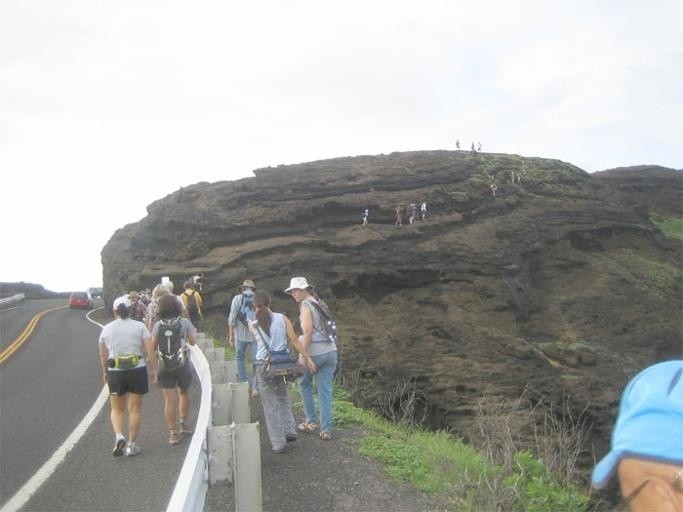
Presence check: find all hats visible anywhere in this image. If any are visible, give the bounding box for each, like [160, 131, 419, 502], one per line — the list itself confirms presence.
[591, 359, 683, 490]
[128, 291, 140, 299]
[284, 277, 313, 295]
[113, 298, 132, 310]
[238, 280, 257, 292]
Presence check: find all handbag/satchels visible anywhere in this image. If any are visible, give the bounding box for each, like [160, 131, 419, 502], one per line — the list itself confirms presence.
[109, 355, 142, 369]
[261, 351, 301, 385]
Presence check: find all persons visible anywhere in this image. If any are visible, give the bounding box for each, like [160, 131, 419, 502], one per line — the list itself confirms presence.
[111, 271, 205, 328]
[513, 168, 527, 185]
[225, 277, 261, 400]
[589, 360, 682, 510]
[246, 288, 318, 454]
[361, 205, 370, 232]
[96, 295, 156, 460]
[392, 199, 428, 226]
[490, 180, 497, 197]
[455, 138, 482, 154]
[147, 295, 197, 447]
[282, 277, 339, 441]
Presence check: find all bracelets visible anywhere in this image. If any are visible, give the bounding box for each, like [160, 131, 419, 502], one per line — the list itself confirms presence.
[102, 368, 108, 373]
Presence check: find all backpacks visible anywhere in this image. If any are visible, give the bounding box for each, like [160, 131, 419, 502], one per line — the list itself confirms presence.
[308, 298, 337, 341]
[238, 293, 256, 325]
[157, 316, 186, 370]
[184, 291, 200, 320]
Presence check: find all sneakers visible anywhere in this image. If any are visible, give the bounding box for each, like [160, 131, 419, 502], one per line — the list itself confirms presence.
[169, 426, 193, 444]
[113, 435, 141, 457]
[273, 423, 332, 453]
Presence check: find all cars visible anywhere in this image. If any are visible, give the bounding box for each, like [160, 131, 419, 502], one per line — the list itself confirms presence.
[68, 290, 95, 310]
